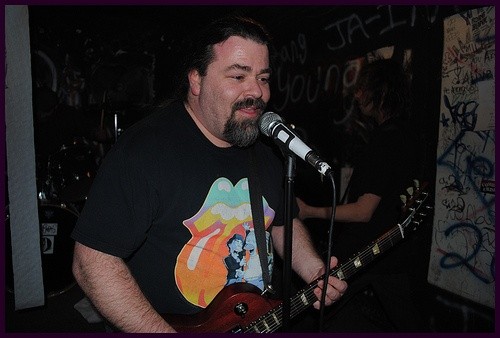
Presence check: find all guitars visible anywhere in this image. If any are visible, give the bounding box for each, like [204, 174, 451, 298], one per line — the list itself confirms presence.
[158, 179, 435, 338]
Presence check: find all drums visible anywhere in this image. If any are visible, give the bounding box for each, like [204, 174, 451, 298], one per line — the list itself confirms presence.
[38, 203, 80, 290]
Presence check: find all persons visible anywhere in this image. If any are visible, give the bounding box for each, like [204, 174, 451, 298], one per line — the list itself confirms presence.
[32, 88, 84, 155]
[295, 59, 420, 324]
[71, 15, 347, 334]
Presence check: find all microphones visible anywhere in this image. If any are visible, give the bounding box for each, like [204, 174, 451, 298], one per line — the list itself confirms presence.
[258, 112, 335, 177]
